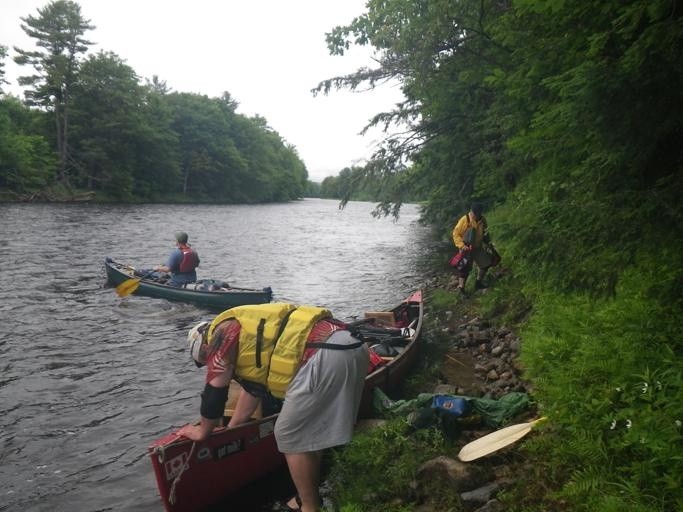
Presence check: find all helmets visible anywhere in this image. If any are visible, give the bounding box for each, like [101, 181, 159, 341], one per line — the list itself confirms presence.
[187, 322, 208, 367]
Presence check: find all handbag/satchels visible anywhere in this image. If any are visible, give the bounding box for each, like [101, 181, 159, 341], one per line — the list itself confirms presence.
[487, 248, 501, 265]
[462, 215, 475, 246]
[449, 253, 473, 272]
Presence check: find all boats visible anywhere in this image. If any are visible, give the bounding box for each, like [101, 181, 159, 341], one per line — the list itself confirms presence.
[148, 289, 423, 511]
[104, 256, 272, 314]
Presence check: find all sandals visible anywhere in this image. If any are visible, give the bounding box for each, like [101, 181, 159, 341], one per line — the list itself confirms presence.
[281, 494, 323, 512]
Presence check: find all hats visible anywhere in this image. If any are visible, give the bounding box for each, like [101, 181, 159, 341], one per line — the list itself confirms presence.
[471, 203, 482, 218]
[174, 232, 188, 243]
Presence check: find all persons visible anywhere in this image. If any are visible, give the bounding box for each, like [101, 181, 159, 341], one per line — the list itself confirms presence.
[452, 202, 492, 294]
[176, 302, 370, 512]
[154, 231, 200, 286]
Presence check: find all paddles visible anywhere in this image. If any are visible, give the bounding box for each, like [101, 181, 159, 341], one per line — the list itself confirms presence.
[458, 416, 547, 462]
[115, 262, 163, 296]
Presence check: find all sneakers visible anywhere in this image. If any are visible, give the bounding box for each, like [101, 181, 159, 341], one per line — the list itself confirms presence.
[475, 280, 486, 288]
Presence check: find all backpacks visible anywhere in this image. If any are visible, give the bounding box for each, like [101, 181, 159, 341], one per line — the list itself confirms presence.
[177, 247, 193, 273]
